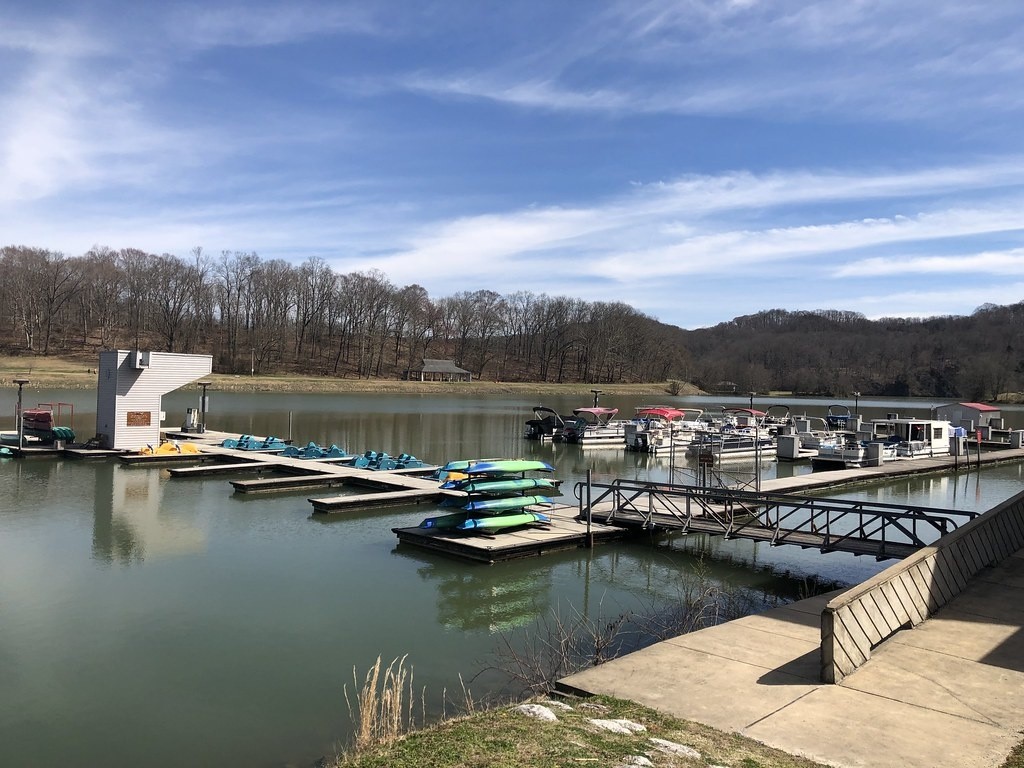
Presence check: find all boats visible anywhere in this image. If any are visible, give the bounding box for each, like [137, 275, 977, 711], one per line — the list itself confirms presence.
[807, 403, 968, 472]
[417, 404, 792, 534]
